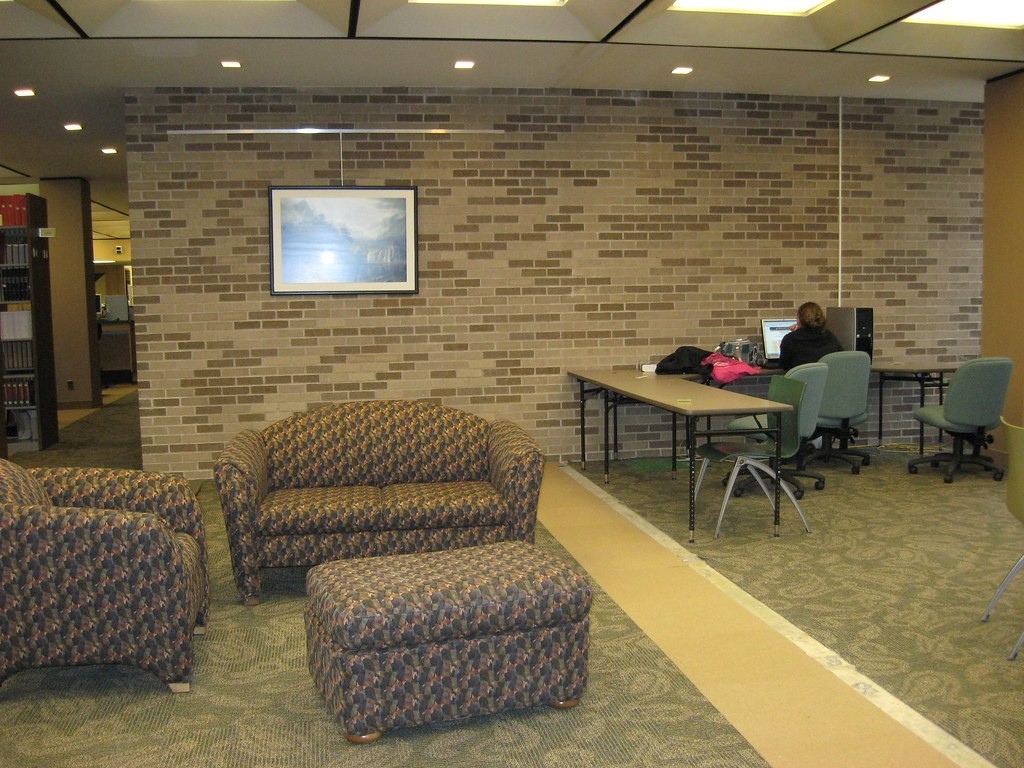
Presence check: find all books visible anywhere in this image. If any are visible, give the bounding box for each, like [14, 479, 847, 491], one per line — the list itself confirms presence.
[0, 195, 32, 404]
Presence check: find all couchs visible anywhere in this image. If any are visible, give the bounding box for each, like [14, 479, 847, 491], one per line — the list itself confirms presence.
[212, 400, 546, 606]
[0, 457, 210, 692]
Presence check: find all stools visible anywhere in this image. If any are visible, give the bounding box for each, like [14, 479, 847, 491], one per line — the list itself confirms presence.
[305, 540, 593, 743]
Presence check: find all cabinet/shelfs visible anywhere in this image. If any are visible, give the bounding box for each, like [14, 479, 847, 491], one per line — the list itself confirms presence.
[0, 192, 58, 453]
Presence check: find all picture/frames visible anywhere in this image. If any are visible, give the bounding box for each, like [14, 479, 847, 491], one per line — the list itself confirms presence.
[267, 185, 419, 296]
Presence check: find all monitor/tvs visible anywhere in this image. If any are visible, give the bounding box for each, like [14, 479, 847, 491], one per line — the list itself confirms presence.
[96, 294, 102, 315]
[762, 319, 798, 360]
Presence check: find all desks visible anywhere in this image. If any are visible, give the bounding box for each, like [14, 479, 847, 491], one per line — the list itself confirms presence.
[566, 366, 790, 542]
[868, 360, 963, 455]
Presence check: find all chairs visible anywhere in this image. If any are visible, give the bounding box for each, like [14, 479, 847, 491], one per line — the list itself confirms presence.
[694, 375, 813, 539]
[907, 356, 1014, 483]
[722, 363, 827, 500]
[796, 351, 870, 474]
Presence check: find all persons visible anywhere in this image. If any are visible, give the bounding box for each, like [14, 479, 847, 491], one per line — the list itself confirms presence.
[780, 301, 843, 370]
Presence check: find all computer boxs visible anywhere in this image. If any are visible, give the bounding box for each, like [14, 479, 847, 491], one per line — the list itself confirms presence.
[826, 306, 873, 366]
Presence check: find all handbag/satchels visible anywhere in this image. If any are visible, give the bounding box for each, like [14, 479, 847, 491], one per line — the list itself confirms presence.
[714, 338, 768, 367]
